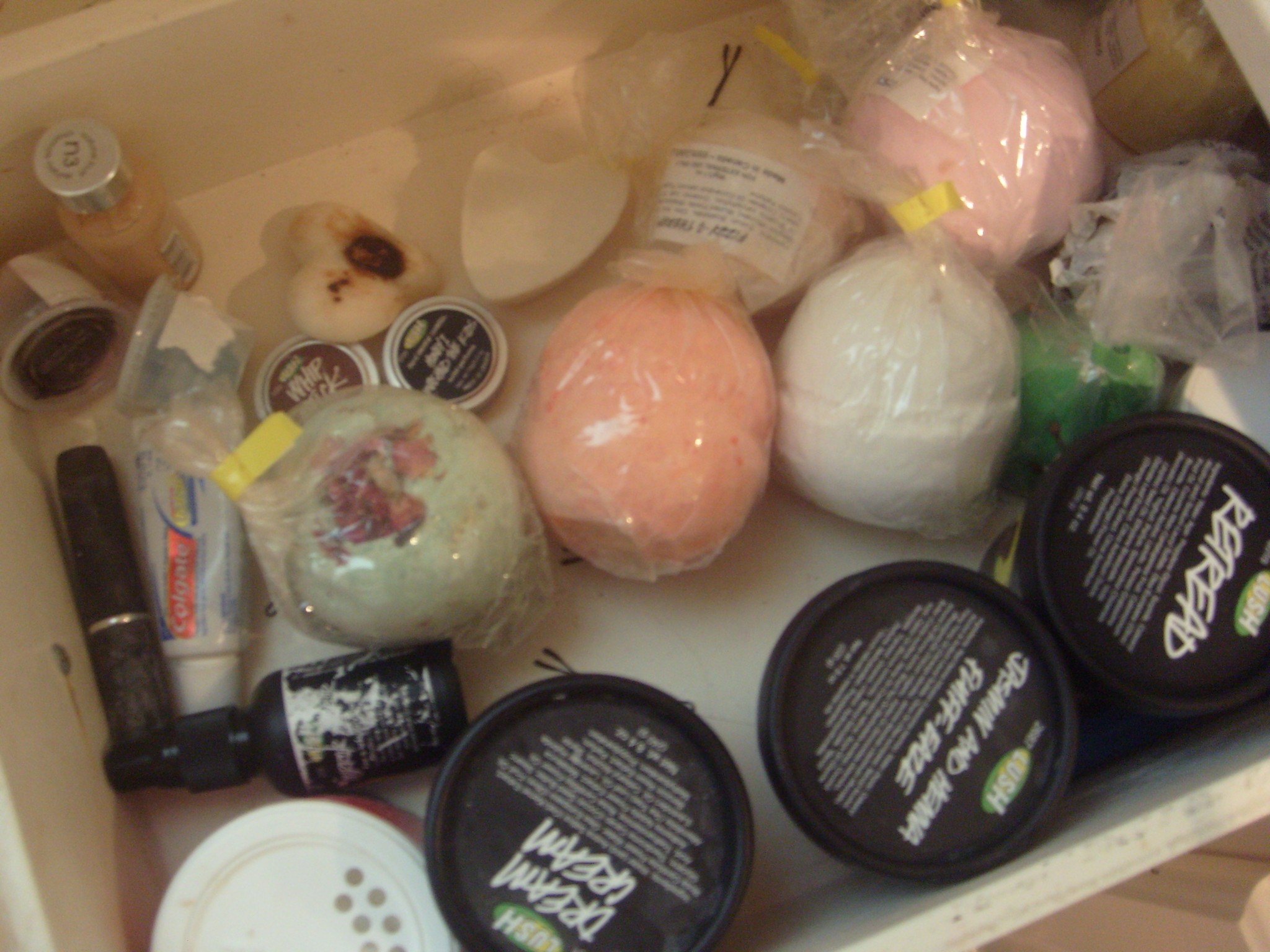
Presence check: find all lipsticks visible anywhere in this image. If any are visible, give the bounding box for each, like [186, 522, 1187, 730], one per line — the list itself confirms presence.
[54, 447, 163, 737]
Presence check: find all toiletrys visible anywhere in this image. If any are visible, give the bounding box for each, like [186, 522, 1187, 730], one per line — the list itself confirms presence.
[133, 405, 254, 712]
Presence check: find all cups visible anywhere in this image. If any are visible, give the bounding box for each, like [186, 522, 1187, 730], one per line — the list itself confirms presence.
[757, 562, 1075, 882]
[147, 798, 463, 952]
[976, 408, 1270, 765]
[1082, 0, 1253, 155]
[421, 673, 753, 952]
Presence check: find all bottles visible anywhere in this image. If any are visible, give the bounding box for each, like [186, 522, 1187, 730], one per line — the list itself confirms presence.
[33, 117, 201, 306]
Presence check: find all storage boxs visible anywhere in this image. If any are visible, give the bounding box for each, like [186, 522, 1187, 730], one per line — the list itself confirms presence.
[1, 0, 1270, 952]
